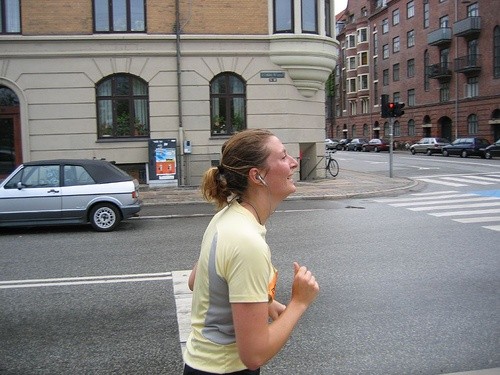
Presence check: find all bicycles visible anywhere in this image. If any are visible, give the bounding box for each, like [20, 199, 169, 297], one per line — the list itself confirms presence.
[325, 151, 339, 177]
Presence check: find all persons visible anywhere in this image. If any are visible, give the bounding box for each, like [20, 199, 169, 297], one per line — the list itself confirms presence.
[184, 128, 319, 375]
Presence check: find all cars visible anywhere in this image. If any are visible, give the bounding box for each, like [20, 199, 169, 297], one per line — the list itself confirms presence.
[410, 137, 451, 156]
[345, 138, 368, 151]
[336, 138, 353, 150]
[0, 158, 144, 233]
[361, 138, 395, 153]
[479, 139, 500, 159]
[442, 137, 491, 158]
[325, 138, 339, 150]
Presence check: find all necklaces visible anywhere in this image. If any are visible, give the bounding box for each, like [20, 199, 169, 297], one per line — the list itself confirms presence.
[241, 201, 262, 224]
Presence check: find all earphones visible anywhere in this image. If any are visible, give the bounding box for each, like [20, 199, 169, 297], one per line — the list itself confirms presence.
[256, 174, 266, 185]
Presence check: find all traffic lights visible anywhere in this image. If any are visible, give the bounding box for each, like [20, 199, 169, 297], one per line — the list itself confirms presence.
[387, 103, 395, 117]
[396, 101, 405, 117]
